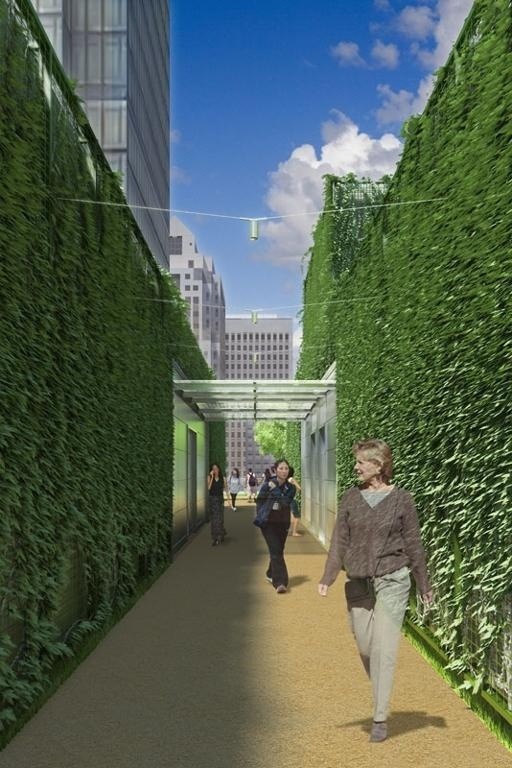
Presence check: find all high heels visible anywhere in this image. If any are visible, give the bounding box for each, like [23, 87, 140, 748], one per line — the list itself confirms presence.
[212, 534, 225, 547]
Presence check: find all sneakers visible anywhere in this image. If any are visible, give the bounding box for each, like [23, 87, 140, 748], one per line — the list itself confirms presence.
[369, 718, 387, 743]
[265, 570, 288, 594]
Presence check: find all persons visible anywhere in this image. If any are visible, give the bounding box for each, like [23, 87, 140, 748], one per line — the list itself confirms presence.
[318, 439, 433, 742]
[229, 468, 241, 512]
[246, 466, 302, 536]
[207, 463, 226, 546]
[256, 459, 296, 593]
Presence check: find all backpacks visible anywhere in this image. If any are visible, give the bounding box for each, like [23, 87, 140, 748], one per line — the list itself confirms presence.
[247, 472, 255, 486]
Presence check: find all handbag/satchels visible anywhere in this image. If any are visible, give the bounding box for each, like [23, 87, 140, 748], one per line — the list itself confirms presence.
[254, 500, 275, 527]
[343, 578, 376, 611]
